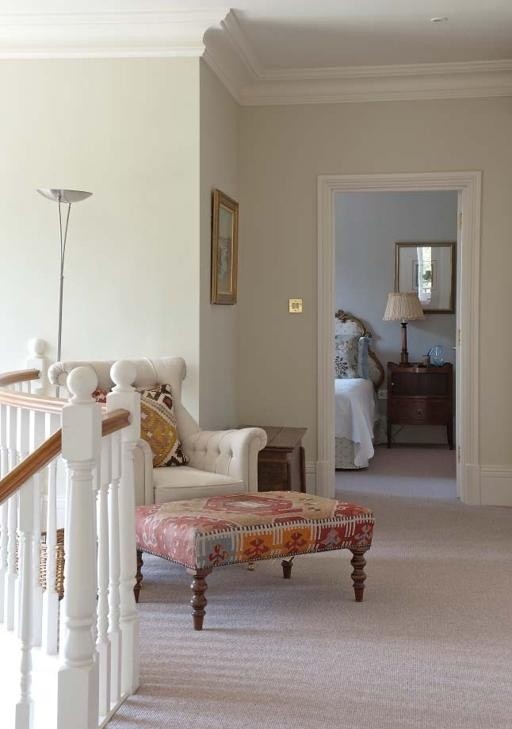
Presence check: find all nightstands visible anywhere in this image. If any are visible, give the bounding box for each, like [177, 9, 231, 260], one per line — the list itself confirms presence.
[386, 359, 454, 449]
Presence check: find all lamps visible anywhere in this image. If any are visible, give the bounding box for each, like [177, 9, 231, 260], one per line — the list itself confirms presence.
[36, 188, 93, 397]
[381, 291, 425, 369]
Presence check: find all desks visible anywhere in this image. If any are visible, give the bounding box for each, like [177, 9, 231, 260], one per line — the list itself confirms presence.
[234, 425, 309, 493]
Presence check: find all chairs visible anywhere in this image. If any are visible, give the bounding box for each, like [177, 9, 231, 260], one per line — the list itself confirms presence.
[48, 356, 270, 510]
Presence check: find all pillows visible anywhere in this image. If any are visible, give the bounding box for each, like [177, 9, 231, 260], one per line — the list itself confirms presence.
[333, 333, 369, 380]
[91, 381, 191, 468]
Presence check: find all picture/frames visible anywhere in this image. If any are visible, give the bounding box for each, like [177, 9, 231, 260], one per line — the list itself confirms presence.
[395, 241, 456, 314]
[208, 187, 241, 307]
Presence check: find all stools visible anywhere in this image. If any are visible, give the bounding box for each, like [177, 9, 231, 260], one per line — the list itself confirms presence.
[134, 488, 376, 632]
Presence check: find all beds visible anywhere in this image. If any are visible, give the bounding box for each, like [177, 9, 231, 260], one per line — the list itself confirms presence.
[333, 309, 385, 472]
